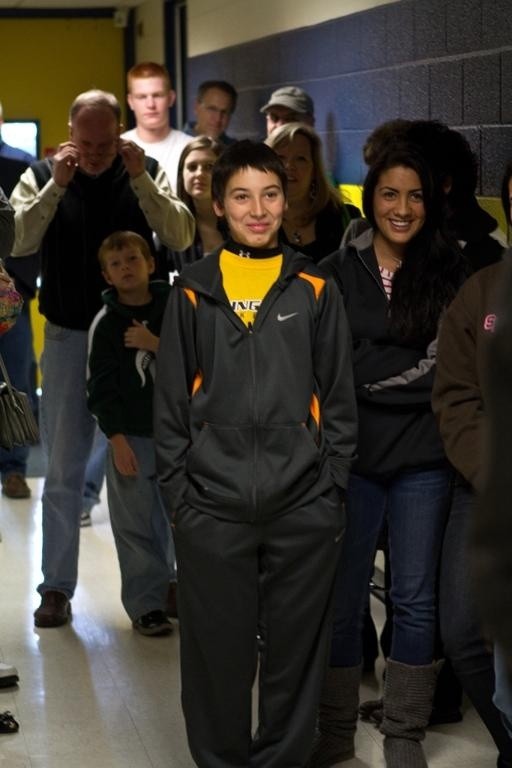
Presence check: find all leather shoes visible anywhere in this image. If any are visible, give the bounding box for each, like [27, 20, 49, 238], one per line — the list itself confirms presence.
[34, 592, 70, 627]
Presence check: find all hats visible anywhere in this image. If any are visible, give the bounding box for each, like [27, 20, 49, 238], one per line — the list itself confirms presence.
[260, 86, 313, 115]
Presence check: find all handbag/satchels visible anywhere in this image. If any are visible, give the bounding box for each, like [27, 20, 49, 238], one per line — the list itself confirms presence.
[1, 378, 40, 451]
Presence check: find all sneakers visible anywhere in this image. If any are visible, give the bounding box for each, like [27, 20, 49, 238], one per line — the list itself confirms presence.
[0, 663, 19, 688]
[360, 689, 463, 725]
[135, 610, 174, 635]
[2, 473, 31, 498]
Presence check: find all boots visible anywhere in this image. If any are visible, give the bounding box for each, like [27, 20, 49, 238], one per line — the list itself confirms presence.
[310, 662, 364, 767]
[383, 658, 443, 768]
